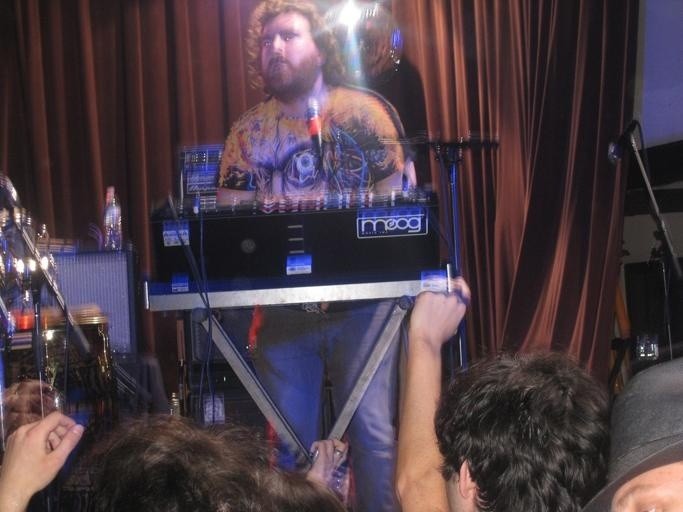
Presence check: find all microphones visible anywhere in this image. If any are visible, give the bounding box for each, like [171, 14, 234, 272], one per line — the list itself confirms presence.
[608, 118, 638, 166]
[307, 97, 323, 168]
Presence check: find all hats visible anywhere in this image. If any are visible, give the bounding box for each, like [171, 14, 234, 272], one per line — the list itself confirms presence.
[582, 355, 682, 507]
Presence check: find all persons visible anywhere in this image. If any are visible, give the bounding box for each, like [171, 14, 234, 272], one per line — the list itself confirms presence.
[394, 264, 683, 512]
[209, 0, 417, 512]
[1, 380, 348, 512]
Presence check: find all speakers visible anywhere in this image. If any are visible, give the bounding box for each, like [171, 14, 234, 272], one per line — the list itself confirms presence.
[624, 258, 683, 374]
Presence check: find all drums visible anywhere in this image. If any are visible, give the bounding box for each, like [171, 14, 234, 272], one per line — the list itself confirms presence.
[1, 206, 58, 307]
[0, 302, 119, 441]
